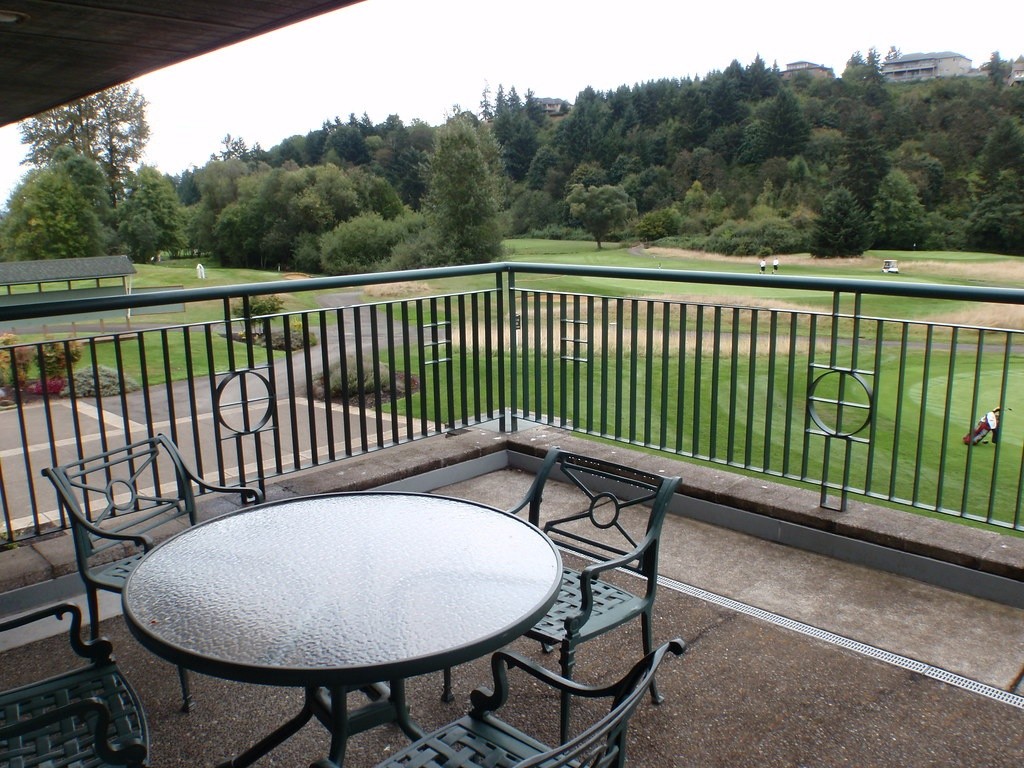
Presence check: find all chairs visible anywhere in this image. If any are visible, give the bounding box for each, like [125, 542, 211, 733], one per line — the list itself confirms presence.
[38, 432, 266, 712]
[309, 636, 689, 767]
[440, 443, 683, 753]
[0, 599, 151, 768]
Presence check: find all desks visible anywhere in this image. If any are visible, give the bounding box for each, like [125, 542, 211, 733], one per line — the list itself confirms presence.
[122, 491, 564, 768]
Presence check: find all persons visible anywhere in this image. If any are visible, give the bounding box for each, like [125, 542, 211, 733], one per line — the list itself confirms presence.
[759, 259, 766, 274]
[771, 258, 779, 275]
